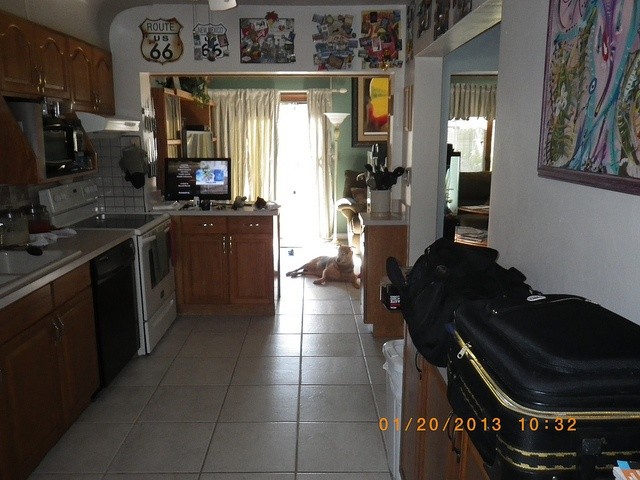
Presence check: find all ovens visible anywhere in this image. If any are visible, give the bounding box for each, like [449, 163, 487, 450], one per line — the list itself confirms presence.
[133, 218, 175, 322]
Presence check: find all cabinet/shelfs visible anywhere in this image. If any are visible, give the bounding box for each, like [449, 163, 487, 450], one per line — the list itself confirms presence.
[419, 361, 490, 479]
[68, 36, 115, 116]
[0, 262, 100, 480]
[0, 9, 70, 101]
[399, 321, 424, 480]
[359, 221, 406, 337]
[0, 97, 98, 184]
[458, 205, 488, 230]
[153, 88, 216, 193]
[173, 210, 274, 317]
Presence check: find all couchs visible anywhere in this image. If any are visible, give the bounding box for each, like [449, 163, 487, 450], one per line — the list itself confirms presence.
[337, 171, 365, 254]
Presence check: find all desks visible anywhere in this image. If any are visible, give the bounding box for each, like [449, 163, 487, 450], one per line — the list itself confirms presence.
[455, 225, 487, 246]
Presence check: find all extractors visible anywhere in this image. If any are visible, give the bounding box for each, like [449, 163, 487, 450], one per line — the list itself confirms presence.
[75, 110, 141, 134]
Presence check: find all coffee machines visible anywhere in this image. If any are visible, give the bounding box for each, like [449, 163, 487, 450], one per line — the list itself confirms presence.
[1, 185, 29, 250]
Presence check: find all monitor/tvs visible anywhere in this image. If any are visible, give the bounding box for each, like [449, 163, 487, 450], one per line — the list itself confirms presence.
[163, 157, 232, 211]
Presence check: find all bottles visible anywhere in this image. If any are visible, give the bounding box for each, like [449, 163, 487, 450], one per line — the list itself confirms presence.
[29, 208, 38, 233]
[39, 206, 49, 233]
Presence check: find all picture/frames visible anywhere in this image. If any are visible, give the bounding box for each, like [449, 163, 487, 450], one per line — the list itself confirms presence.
[352, 77, 389, 148]
[536, 0, 640, 197]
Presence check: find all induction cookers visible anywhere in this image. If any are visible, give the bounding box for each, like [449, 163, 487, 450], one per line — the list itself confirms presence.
[39, 176, 169, 236]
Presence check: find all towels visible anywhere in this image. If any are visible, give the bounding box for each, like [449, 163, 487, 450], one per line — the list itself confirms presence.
[151, 230, 169, 281]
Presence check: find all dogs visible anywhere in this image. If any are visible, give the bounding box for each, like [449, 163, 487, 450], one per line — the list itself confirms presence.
[286, 243, 361, 289]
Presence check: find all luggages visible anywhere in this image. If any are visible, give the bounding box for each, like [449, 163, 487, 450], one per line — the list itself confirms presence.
[447, 294, 640, 480]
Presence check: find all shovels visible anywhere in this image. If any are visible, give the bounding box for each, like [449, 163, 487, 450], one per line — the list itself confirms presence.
[365, 175, 377, 191]
[362, 162, 374, 175]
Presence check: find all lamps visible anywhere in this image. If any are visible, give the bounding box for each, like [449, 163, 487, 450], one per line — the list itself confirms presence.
[323, 112, 350, 240]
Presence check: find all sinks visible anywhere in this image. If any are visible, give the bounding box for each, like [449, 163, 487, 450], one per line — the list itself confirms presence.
[0, 275, 20, 288]
[0, 247, 63, 275]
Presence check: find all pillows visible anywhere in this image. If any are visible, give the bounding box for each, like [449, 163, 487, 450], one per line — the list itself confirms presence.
[351, 188, 367, 209]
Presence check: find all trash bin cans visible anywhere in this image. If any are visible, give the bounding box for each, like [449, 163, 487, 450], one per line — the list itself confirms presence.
[383, 339, 405, 480]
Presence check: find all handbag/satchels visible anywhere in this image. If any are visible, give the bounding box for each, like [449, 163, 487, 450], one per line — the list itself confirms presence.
[386, 238, 532, 367]
